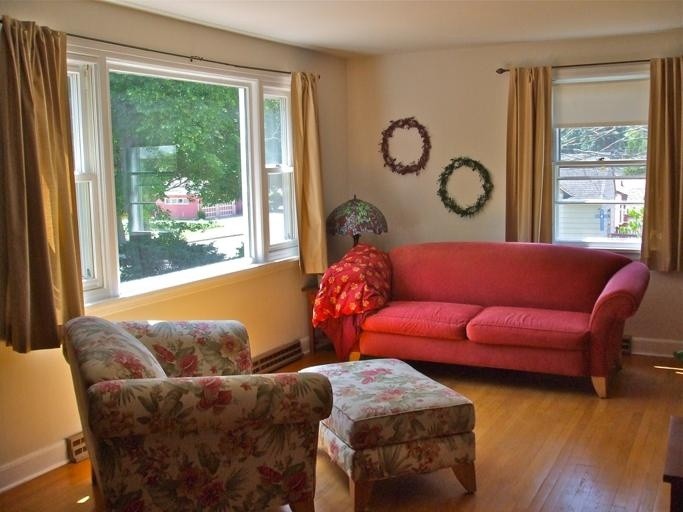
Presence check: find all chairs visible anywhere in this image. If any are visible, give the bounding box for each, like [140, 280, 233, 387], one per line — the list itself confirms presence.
[63, 317, 331, 512]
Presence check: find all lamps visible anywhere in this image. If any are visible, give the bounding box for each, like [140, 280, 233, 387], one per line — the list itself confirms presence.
[325, 195, 387, 248]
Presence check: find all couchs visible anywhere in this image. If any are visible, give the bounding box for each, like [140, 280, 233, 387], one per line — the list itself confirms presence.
[313, 240, 649, 399]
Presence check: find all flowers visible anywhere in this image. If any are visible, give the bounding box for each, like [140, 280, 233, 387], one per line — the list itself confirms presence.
[379, 117, 430, 177]
[436, 155, 492, 218]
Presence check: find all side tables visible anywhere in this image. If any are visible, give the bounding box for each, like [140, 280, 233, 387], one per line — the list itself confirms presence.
[302, 279, 333, 358]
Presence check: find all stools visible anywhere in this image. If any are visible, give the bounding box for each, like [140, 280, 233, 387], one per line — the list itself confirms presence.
[294, 358, 476, 511]
